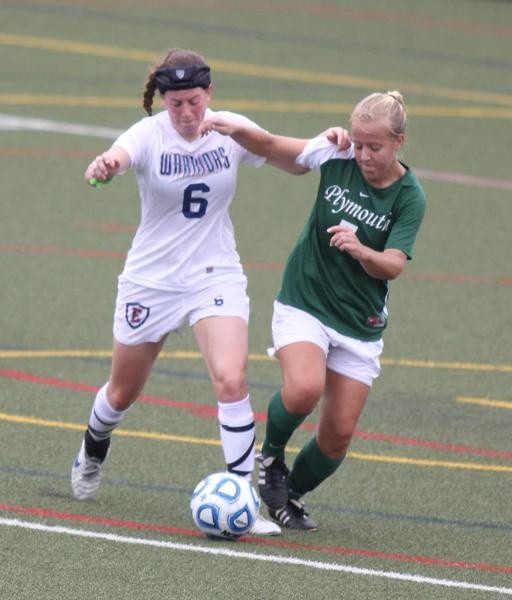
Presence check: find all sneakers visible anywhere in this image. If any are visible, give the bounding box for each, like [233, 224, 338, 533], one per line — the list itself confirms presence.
[247, 454, 318, 535]
[71, 440, 112, 500]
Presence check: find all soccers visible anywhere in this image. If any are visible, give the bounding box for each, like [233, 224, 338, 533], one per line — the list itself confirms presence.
[191, 473, 259, 538]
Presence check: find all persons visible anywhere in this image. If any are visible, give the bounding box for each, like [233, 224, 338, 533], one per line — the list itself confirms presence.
[198, 89, 427, 533]
[70, 47, 351, 536]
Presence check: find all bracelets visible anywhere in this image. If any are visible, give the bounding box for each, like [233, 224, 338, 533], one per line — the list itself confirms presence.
[89, 175, 114, 188]
